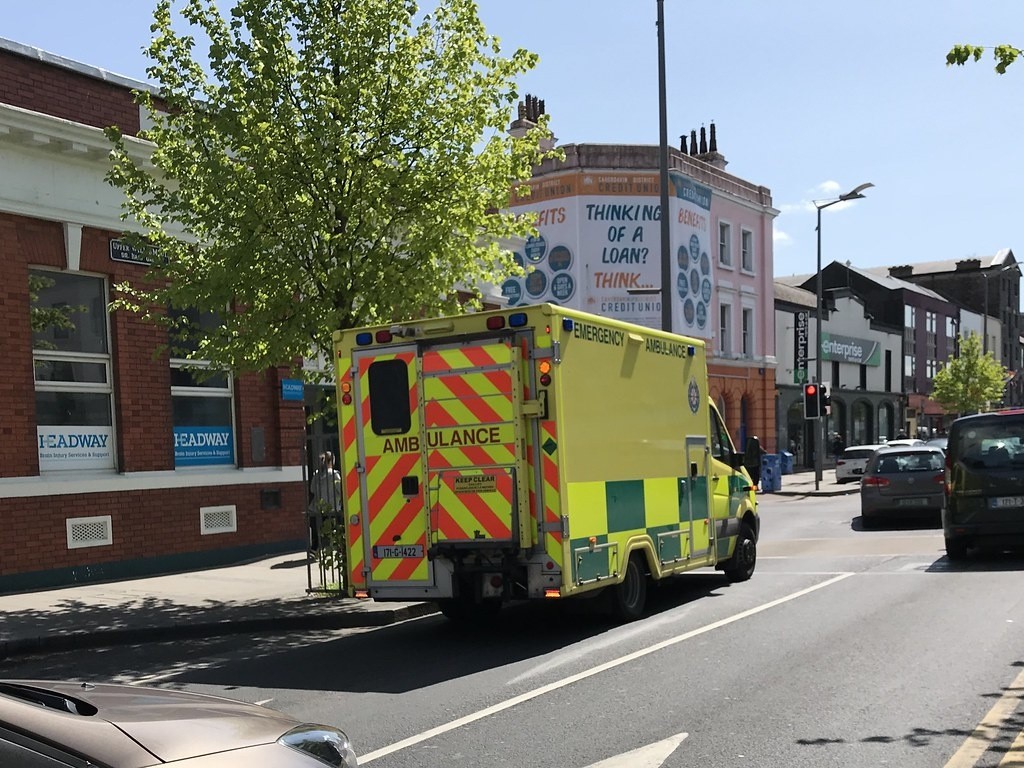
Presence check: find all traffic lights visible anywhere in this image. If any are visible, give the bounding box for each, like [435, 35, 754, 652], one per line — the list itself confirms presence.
[804, 383, 820, 419]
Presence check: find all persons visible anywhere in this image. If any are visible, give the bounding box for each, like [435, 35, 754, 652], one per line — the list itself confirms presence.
[749, 435, 767, 491]
[309, 451, 341, 560]
[832, 426, 947, 465]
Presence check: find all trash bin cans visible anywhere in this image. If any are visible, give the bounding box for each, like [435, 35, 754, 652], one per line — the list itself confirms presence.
[779, 449, 794, 475]
[760, 453, 782, 491]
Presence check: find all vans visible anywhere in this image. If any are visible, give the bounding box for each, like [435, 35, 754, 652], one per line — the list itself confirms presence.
[943, 409, 1024, 558]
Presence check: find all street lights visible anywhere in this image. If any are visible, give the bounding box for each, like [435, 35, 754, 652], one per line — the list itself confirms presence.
[984, 261, 1023, 356]
[811, 182, 875, 480]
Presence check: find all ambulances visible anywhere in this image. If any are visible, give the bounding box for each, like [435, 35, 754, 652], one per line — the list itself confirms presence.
[331, 302, 762, 623]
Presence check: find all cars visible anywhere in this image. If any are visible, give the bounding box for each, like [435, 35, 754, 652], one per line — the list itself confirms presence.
[888, 439, 928, 449]
[861, 446, 947, 528]
[835, 445, 890, 483]
[0, 678, 358, 768]
[926, 438, 948, 453]
